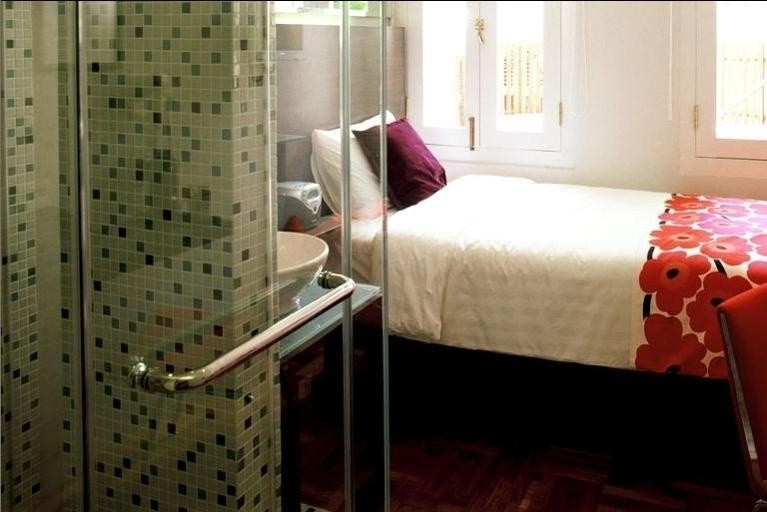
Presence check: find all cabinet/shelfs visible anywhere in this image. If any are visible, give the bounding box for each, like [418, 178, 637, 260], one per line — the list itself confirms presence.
[280, 283, 391, 512]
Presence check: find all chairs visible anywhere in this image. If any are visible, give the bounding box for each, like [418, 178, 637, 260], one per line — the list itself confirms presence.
[715, 273, 766, 512]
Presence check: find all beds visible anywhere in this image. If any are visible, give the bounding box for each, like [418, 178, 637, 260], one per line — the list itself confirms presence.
[282, 28, 767, 391]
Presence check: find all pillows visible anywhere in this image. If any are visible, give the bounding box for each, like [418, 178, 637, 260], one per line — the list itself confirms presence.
[352, 116, 451, 211]
[303, 108, 412, 223]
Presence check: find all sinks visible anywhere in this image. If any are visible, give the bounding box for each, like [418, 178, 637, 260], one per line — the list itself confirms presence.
[277, 231, 329, 314]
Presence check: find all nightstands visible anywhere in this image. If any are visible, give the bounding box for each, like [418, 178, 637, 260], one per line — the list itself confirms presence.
[302, 216, 345, 261]
